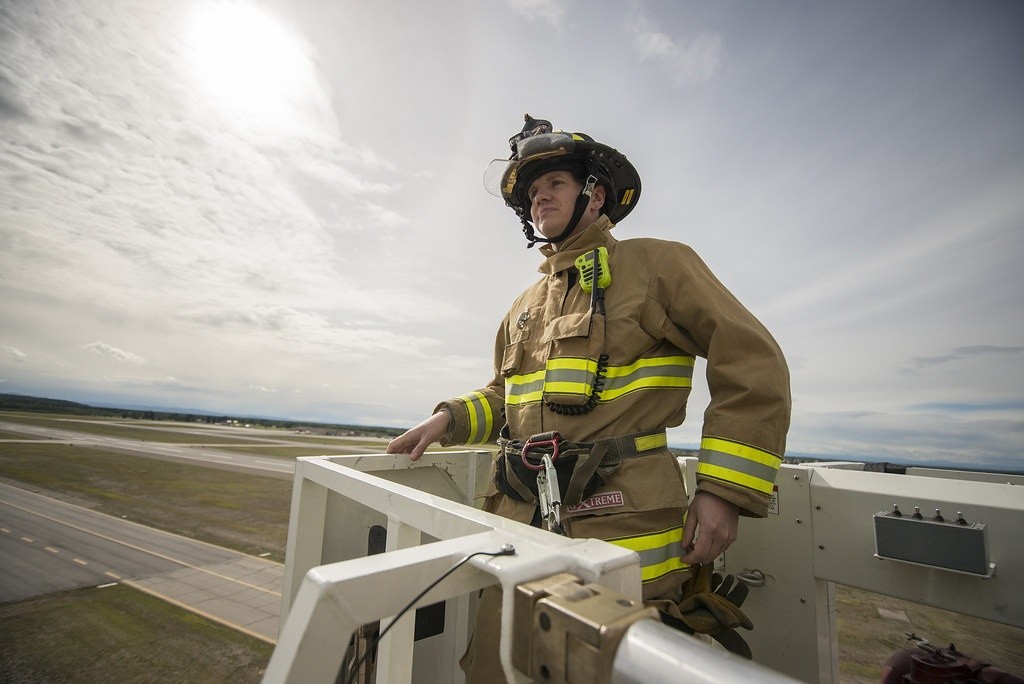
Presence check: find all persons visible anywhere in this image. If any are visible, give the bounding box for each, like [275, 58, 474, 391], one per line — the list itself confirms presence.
[387, 133, 790, 637]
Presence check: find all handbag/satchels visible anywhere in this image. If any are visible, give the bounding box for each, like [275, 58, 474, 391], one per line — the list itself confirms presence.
[493, 438, 594, 504]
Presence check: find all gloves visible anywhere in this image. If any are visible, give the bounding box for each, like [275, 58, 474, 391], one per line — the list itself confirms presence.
[679, 560, 753, 659]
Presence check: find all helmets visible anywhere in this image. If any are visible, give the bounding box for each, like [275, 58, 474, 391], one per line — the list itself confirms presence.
[482, 116, 641, 239]
